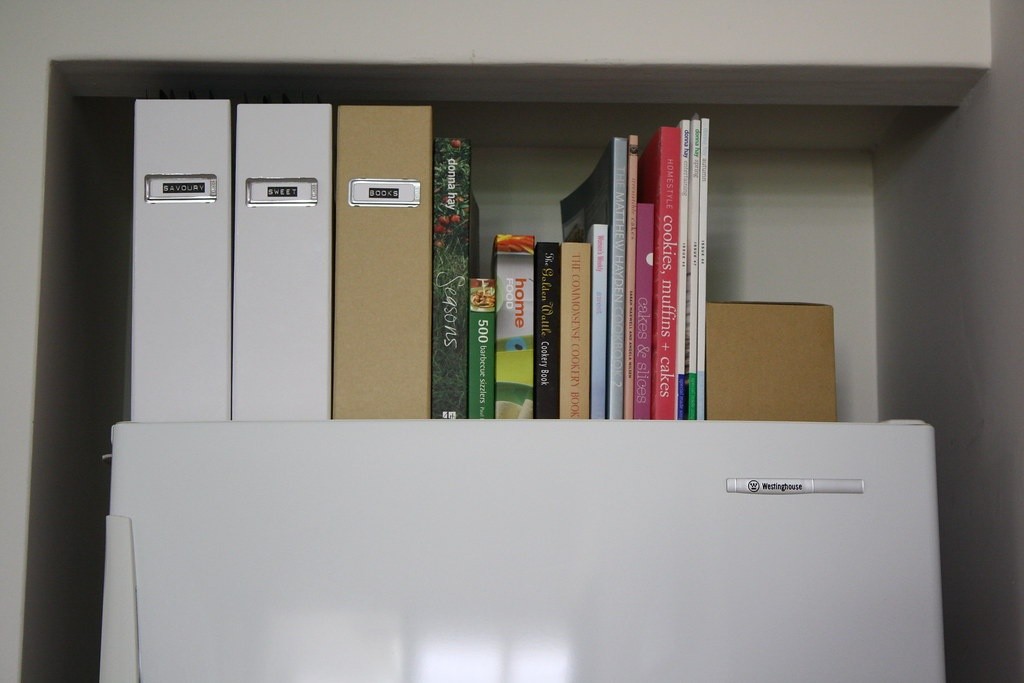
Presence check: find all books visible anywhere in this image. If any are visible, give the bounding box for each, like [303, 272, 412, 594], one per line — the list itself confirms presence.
[431, 117, 710, 421]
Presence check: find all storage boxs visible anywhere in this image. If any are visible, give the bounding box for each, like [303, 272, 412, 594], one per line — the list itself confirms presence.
[705, 299, 840, 423]
